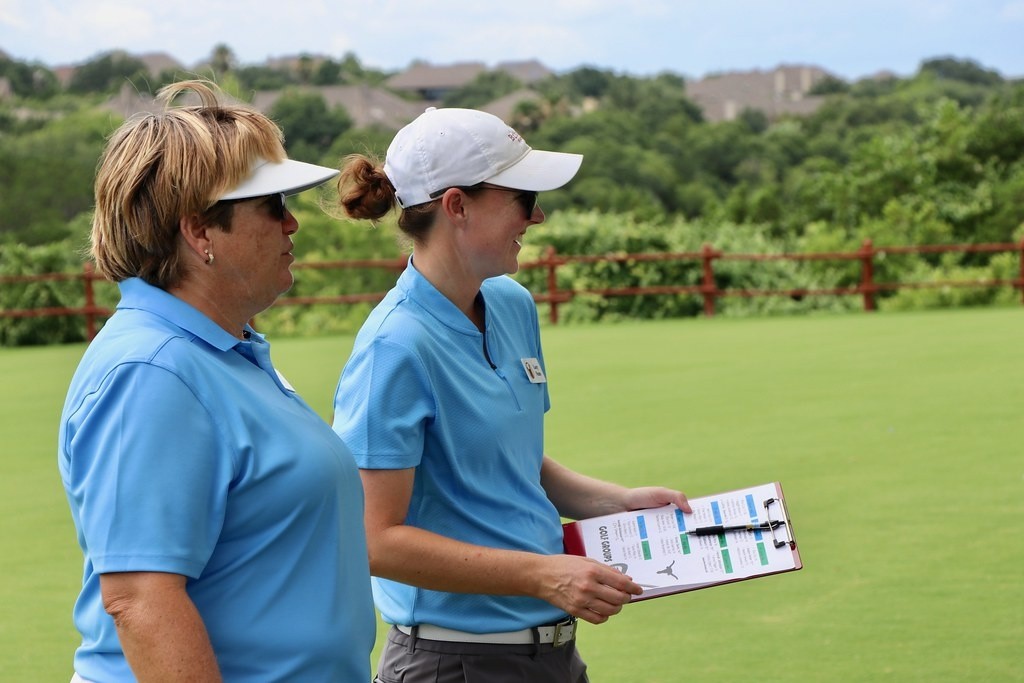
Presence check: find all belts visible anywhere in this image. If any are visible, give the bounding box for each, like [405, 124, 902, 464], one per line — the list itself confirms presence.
[396, 618, 578, 648]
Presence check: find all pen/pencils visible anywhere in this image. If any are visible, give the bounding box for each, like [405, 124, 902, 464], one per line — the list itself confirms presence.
[685, 520, 786, 536]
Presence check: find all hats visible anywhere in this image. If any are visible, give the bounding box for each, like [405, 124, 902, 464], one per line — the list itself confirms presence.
[383, 106, 585, 210]
[208, 152, 340, 202]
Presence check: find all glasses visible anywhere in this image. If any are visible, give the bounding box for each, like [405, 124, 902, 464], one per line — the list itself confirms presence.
[429, 185, 539, 220]
[223, 193, 286, 221]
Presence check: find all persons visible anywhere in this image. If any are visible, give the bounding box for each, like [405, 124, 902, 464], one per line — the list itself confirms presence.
[57, 80, 375, 683]
[331, 106, 692, 683]
